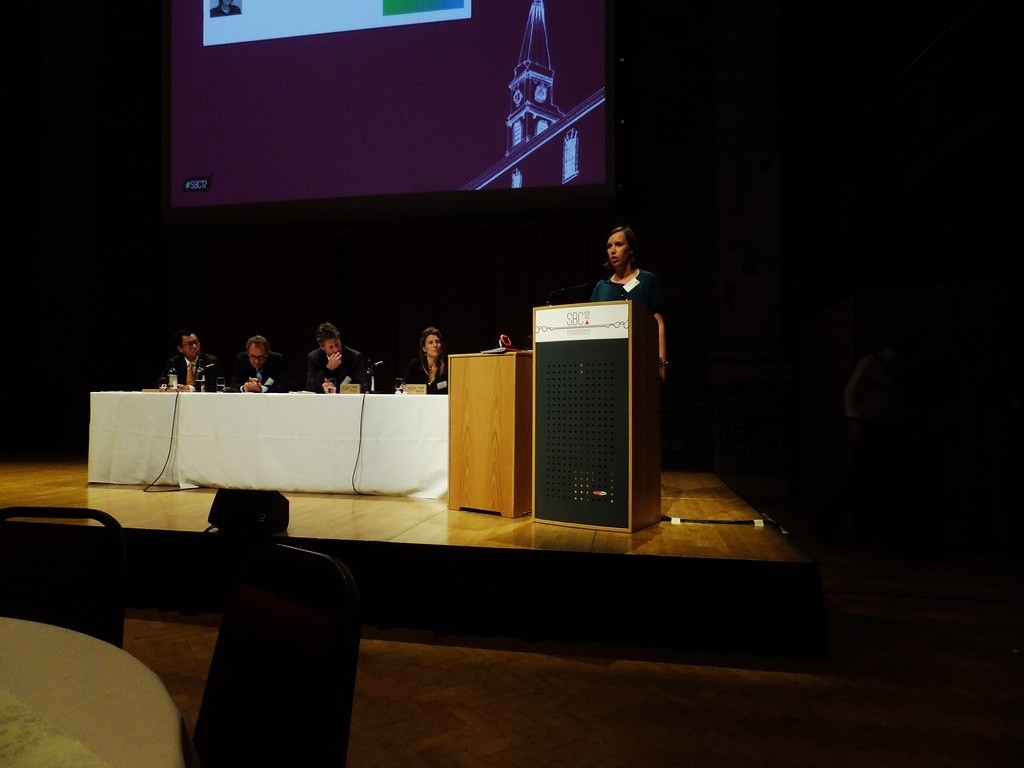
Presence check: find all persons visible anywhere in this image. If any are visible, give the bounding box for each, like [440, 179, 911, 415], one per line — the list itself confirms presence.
[587, 224, 672, 384]
[404, 327, 449, 394]
[225, 334, 289, 393]
[161, 330, 224, 392]
[306, 322, 370, 393]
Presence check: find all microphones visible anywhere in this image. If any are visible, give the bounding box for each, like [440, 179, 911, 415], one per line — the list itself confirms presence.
[544, 280, 597, 307]
[201, 363, 214, 371]
[366, 360, 383, 374]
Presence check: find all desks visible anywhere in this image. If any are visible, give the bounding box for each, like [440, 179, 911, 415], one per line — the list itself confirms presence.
[87, 392, 449, 499]
[0, 616, 201, 768]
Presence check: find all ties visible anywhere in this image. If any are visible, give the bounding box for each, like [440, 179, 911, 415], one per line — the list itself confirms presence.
[187, 363, 195, 386]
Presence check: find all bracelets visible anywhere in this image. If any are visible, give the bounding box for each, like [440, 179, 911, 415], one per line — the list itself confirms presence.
[658, 359, 671, 368]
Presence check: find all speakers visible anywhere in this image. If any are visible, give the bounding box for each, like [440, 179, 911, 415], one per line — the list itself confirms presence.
[207, 488, 289, 537]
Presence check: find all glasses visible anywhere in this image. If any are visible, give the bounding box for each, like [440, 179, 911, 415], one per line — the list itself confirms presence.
[183, 339, 199, 347]
[246, 352, 266, 360]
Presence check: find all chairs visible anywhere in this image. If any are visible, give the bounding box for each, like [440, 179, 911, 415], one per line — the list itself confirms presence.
[0, 506, 362, 768]
